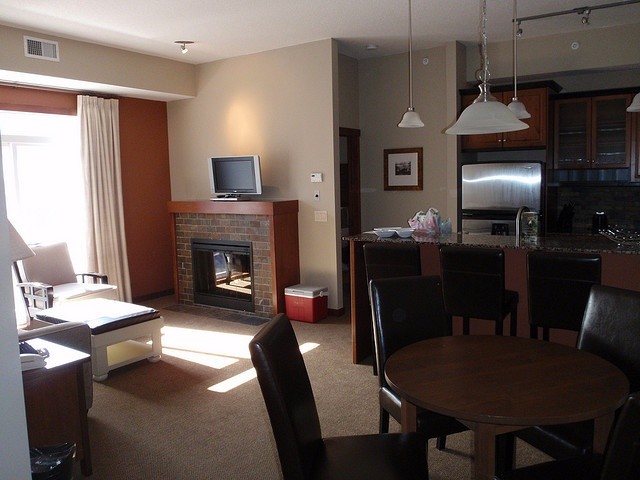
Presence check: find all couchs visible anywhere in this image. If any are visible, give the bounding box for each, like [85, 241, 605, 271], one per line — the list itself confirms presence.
[18, 323, 94, 413]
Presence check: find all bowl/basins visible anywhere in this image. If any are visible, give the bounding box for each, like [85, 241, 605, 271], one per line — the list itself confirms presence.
[373, 230, 397, 238]
[396, 230, 415, 237]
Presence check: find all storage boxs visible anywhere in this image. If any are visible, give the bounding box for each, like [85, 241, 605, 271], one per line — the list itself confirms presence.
[283, 284, 328, 323]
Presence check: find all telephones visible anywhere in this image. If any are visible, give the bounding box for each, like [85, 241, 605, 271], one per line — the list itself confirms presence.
[19, 349, 50, 372]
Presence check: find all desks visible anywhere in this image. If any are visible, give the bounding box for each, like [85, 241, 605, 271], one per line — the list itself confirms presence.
[386, 333, 631, 480]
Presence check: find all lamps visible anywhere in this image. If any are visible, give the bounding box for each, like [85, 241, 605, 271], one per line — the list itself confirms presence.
[396, 0, 425, 128]
[444, 0, 530, 135]
[504, 1, 531, 120]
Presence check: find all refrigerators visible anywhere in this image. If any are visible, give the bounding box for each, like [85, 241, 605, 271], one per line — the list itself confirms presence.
[458, 161, 545, 236]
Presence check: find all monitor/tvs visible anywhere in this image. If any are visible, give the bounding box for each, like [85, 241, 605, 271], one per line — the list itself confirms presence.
[208, 154, 263, 201]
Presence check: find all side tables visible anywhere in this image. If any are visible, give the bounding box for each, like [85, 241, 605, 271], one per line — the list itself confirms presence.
[18, 338, 94, 476]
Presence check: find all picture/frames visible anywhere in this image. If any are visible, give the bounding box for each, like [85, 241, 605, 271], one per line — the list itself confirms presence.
[383, 147, 424, 192]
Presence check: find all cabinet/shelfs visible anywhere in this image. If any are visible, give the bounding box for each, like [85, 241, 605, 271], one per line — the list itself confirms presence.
[463, 89, 554, 149]
[553, 95, 631, 167]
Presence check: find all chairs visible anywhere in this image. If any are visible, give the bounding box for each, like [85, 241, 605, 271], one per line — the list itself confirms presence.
[439, 244, 519, 336]
[578, 287, 640, 480]
[14, 240, 119, 323]
[526, 252, 601, 340]
[248, 312, 427, 480]
[363, 243, 422, 375]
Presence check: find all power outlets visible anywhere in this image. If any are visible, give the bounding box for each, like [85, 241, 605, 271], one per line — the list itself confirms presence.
[314, 190, 319, 201]
